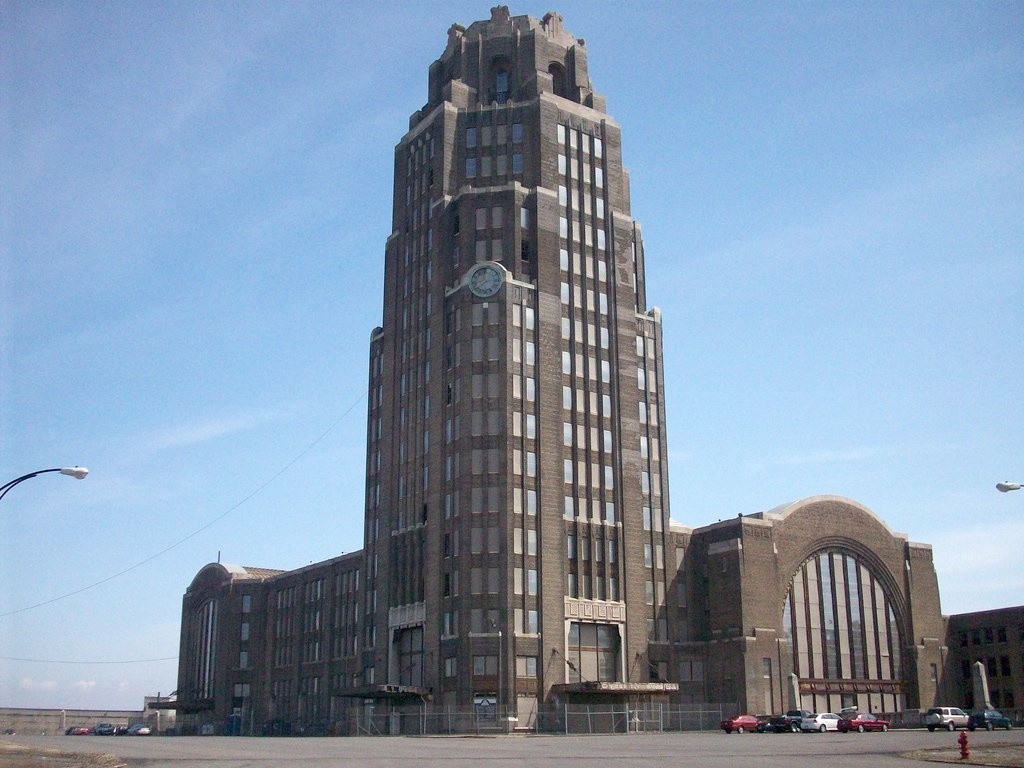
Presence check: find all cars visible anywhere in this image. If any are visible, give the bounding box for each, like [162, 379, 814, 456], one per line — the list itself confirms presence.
[720, 714, 779, 734]
[67, 726, 90, 735]
[116, 724, 151, 735]
[968, 710, 1013, 732]
[801, 713, 843, 733]
[838, 712, 889, 733]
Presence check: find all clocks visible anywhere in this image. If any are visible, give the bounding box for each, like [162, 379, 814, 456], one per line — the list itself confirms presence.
[468, 264, 503, 298]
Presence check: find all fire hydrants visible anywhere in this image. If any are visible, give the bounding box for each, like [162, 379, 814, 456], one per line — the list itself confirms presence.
[957, 729, 970, 758]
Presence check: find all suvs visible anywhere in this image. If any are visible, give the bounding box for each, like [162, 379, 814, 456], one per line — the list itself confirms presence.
[95, 723, 116, 736]
[925, 707, 969, 732]
[770, 710, 811, 733]
